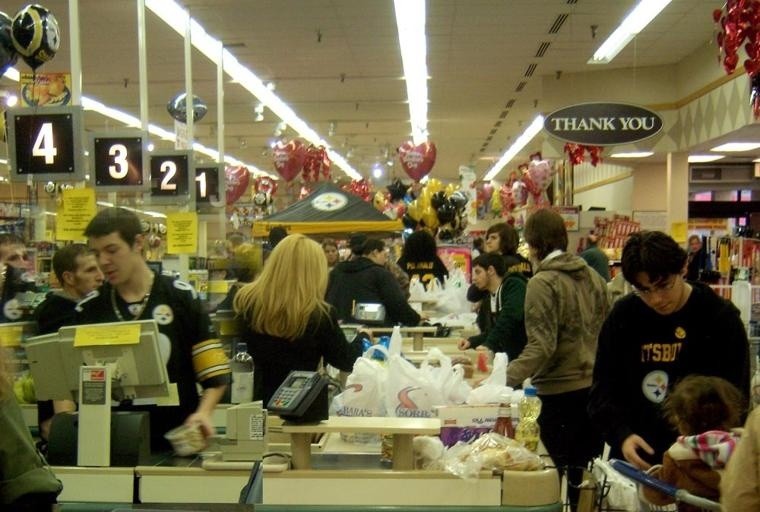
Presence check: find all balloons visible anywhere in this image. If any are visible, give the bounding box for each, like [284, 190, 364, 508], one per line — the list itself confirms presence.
[252, 193, 273, 209]
[166, 91, 207, 121]
[222, 163, 248, 204]
[256, 177, 276, 195]
[341, 155, 552, 240]
[13, 5, 61, 70]
[400, 141, 436, 180]
[1, 12, 19, 75]
[270, 137, 305, 182]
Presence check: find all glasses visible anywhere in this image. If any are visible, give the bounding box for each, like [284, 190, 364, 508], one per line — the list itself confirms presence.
[630, 275, 676, 297]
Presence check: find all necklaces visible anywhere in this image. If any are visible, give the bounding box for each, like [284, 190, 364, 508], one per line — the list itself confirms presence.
[109, 283, 153, 323]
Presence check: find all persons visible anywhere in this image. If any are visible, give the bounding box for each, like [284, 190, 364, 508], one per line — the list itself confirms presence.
[579, 234, 611, 283]
[0, 233, 45, 320]
[639, 375, 744, 511]
[29, 242, 106, 465]
[228, 227, 430, 424]
[682, 235, 711, 286]
[505, 208, 615, 510]
[584, 229, 751, 473]
[60, 206, 231, 459]
[458, 223, 534, 363]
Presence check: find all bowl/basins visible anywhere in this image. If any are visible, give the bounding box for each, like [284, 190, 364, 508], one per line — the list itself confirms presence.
[164, 422, 206, 457]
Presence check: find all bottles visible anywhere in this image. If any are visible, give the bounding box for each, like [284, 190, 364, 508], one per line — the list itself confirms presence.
[514, 387, 542, 451]
[440, 417, 463, 450]
[231, 343, 254, 405]
[494, 394, 514, 439]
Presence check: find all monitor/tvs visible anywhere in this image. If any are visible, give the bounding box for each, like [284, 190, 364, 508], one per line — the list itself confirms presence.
[24, 319, 171, 403]
